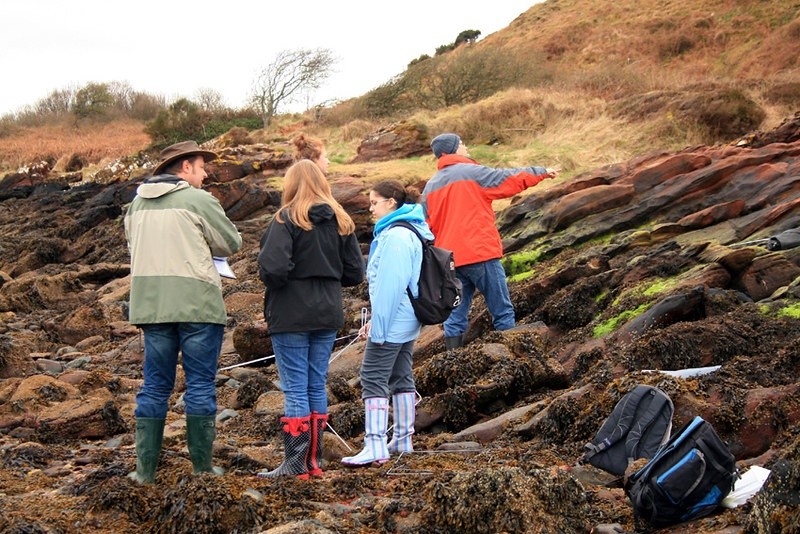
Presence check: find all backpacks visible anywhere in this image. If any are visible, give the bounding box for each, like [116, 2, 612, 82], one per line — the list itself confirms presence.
[576, 384, 674, 478]
[388, 221, 463, 325]
[623, 416, 742, 534]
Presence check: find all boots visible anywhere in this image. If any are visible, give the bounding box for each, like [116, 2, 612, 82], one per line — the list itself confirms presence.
[387, 392, 415, 456]
[127, 417, 166, 488]
[341, 398, 390, 467]
[257, 417, 311, 480]
[186, 415, 224, 477]
[307, 411, 328, 475]
[445, 334, 464, 351]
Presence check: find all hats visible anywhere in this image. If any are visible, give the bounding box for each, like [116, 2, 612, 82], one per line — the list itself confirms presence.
[153, 140, 218, 176]
[430, 133, 460, 158]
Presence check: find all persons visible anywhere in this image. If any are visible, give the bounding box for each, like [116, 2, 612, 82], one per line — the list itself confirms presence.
[255, 158, 365, 480]
[419, 132, 561, 351]
[341, 180, 462, 464]
[293, 135, 329, 173]
[123, 141, 243, 485]
